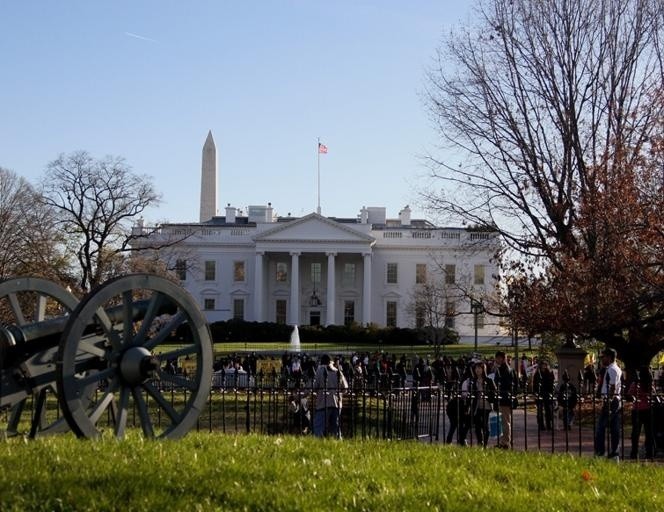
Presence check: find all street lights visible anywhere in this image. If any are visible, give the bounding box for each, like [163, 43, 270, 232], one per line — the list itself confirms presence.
[468, 293, 484, 353]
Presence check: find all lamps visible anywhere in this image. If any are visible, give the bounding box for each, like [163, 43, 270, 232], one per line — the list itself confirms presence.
[310, 258, 318, 307]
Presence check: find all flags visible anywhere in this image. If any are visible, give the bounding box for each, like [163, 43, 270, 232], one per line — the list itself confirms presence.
[318, 142, 328, 154]
